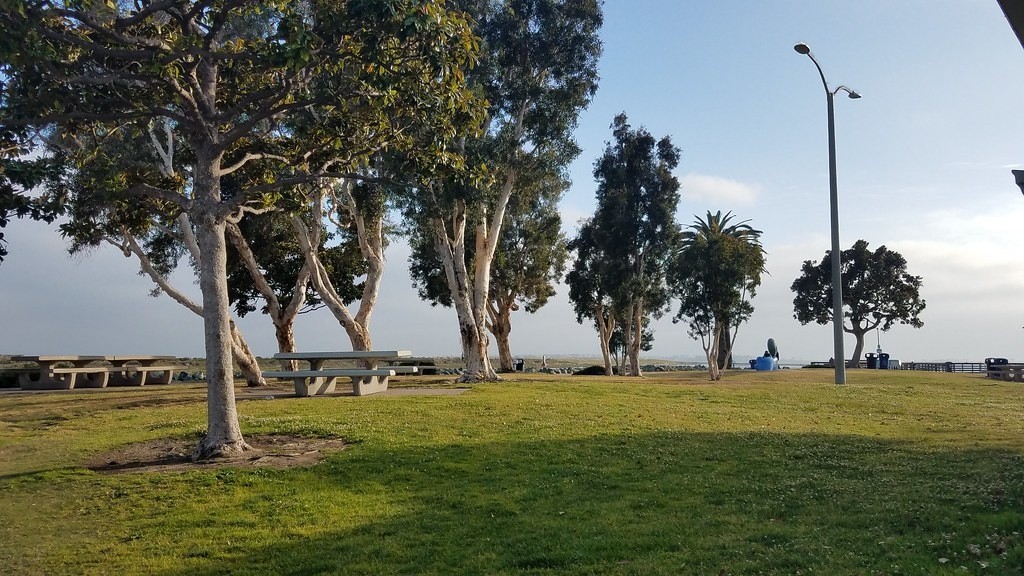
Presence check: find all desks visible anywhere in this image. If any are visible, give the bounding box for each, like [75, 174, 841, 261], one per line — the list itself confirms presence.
[388, 357, 434, 366]
[988, 365, 1024, 381]
[115, 355, 176, 387]
[274, 350, 412, 397]
[11, 355, 114, 390]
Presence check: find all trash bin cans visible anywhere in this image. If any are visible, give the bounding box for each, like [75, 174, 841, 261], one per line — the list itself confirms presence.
[864, 353, 879, 369]
[945, 362, 953, 372]
[985, 357, 1008, 377]
[516, 358, 524, 371]
[879, 353, 889, 369]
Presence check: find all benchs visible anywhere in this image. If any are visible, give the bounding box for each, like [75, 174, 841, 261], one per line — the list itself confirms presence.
[299, 366, 418, 373]
[261, 369, 396, 378]
[48, 366, 127, 373]
[413, 365, 436, 375]
[127, 365, 188, 372]
[984, 370, 1024, 375]
[0, 367, 64, 377]
